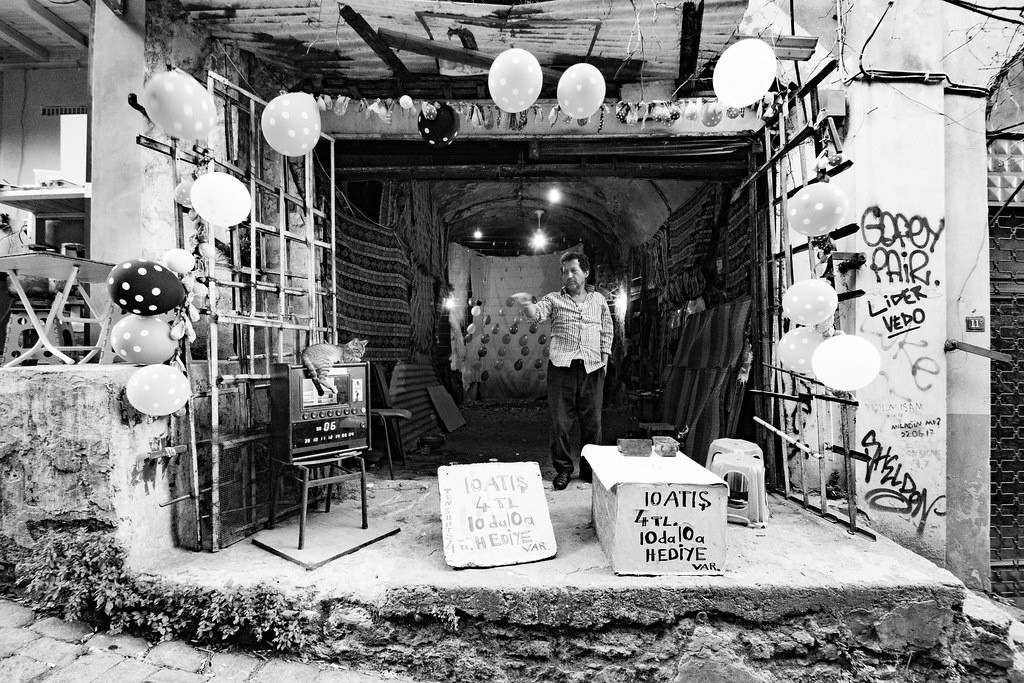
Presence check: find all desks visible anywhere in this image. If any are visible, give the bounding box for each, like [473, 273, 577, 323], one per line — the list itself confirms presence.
[592, 467, 726, 574]
[0, 251, 117, 367]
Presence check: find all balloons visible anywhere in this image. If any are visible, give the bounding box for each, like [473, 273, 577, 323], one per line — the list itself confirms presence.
[464, 296, 550, 383]
[261, 92, 321, 157]
[713, 38, 777, 108]
[788, 182, 849, 236]
[106, 70, 252, 415]
[488, 48, 543, 113]
[418, 102, 461, 147]
[777, 279, 881, 391]
[557, 63, 606, 119]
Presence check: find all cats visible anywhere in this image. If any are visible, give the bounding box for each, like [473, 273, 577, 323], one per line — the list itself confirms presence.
[301, 338, 368, 396]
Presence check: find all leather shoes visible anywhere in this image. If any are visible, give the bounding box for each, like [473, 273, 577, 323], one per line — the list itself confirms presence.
[552, 470, 571, 489]
[578, 471, 592, 482]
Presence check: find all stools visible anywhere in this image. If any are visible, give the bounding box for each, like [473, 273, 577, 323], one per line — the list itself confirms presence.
[705, 437, 769, 528]
[371, 409, 412, 479]
[2, 312, 79, 365]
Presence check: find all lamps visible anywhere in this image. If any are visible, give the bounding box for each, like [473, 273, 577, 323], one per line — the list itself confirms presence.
[527, 210, 554, 254]
[473, 227, 483, 240]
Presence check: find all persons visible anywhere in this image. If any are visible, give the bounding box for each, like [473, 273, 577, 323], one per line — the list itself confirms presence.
[515, 252, 614, 490]
